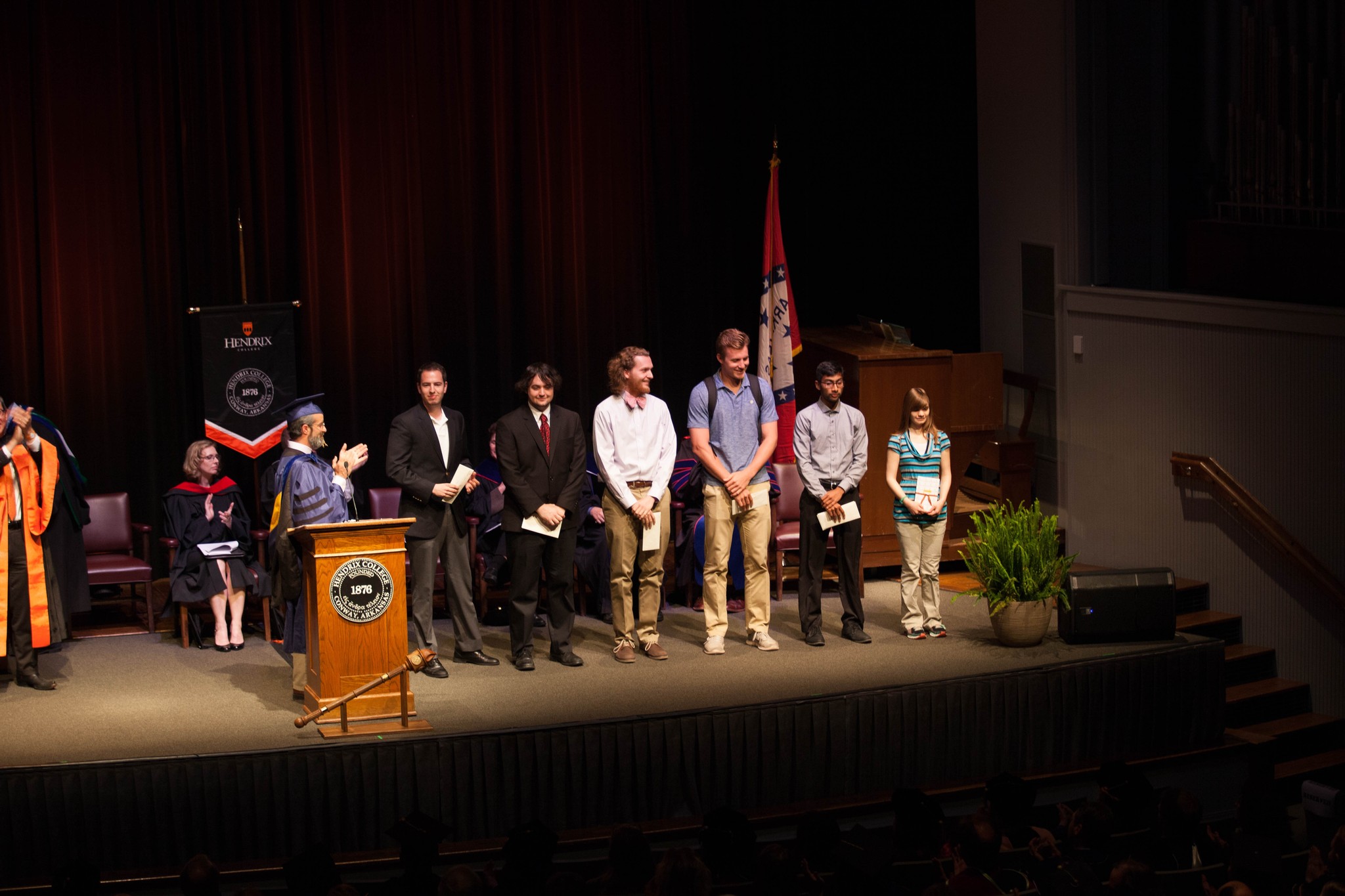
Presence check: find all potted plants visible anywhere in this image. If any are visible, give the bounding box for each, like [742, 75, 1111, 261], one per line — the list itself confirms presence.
[951, 497, 1080, 648]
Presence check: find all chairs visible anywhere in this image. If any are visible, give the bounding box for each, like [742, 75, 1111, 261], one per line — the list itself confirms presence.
[368, 488, 480, 606]
[770, 463, 864, 601]
[476, 553, 509, 619]
[159, 530, 272, 649]
[83, 493, 154, 634]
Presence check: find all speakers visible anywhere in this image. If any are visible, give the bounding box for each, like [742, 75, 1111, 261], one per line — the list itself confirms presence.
[1057, 567, 1177, 644]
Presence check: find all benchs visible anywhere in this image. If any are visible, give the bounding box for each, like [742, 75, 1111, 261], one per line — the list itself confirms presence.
[959, 369, 1037, 520]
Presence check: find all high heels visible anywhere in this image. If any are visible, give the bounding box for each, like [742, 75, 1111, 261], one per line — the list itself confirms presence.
[214, 628, 244, 651]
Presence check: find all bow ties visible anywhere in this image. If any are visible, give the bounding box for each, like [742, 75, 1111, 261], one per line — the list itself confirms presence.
[625, 393, 646, 409]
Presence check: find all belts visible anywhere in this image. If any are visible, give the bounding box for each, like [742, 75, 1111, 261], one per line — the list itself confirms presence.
[822, 481, 838, 490]
[627, 481, 652, 489]
[8, 522, 21, 531]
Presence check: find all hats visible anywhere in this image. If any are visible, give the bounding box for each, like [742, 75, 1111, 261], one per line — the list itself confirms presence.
[271, 393, 325, 425]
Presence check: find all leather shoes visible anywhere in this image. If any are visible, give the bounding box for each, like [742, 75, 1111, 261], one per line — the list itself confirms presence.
[533, 613, 546, 626]
[482, 566, 499, 584]
[17, 673, 57, 690]
[693, 595, 745, 613]
[423, 648, 585, 678]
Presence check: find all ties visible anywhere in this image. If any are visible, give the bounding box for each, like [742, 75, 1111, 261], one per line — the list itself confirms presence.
[539, 414, 550, 460]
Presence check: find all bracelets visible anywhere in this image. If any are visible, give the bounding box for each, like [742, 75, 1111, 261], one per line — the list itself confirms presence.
[899, 496, 907, 504]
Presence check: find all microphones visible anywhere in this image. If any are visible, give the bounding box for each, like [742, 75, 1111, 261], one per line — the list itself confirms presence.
[344, 461, 359, 521]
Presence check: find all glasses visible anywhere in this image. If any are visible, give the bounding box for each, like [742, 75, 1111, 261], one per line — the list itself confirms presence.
[200, 455, 221, 462]
[821, 380, 845, 389]
[309, 422, 324, 430]
[0, 410, 5, 416]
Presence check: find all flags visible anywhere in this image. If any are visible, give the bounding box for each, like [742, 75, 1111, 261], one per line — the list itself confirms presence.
[758, 160, 810, 464]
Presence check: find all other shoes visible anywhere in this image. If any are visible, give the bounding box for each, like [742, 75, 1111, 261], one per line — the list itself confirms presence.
[604, 613, 613, 623]
[292, 689, 305, 700]
[657, 610, 664, 621]
[38, 642, 62, 655]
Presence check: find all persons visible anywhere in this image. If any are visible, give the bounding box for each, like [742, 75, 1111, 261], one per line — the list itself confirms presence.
[584, 471, 664, 624]
[793, 362, 872, 647]
[264, 391, 368, 701]
[471, 423, 546, 626]
[886, 387, 952, 639]
[688, 329, 780, 654]
[0, 398, 92, 692]
[387, 362, 500, 678]
[495, 363, 585, 670]
[161, 440, 251, 652]
[593, 347, 677, 662]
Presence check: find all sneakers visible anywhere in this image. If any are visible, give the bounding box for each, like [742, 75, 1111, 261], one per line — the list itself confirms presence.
[703, 635, 725, 654]
[746, 630, 779, 650]
[928, 624, 947, 637]
[842, 623, 872, 643]
[903, 626, 926, 639]
[806, 626, 825, 646]
[639, 641, 668, 660]
[613, 638, 636, 662]
